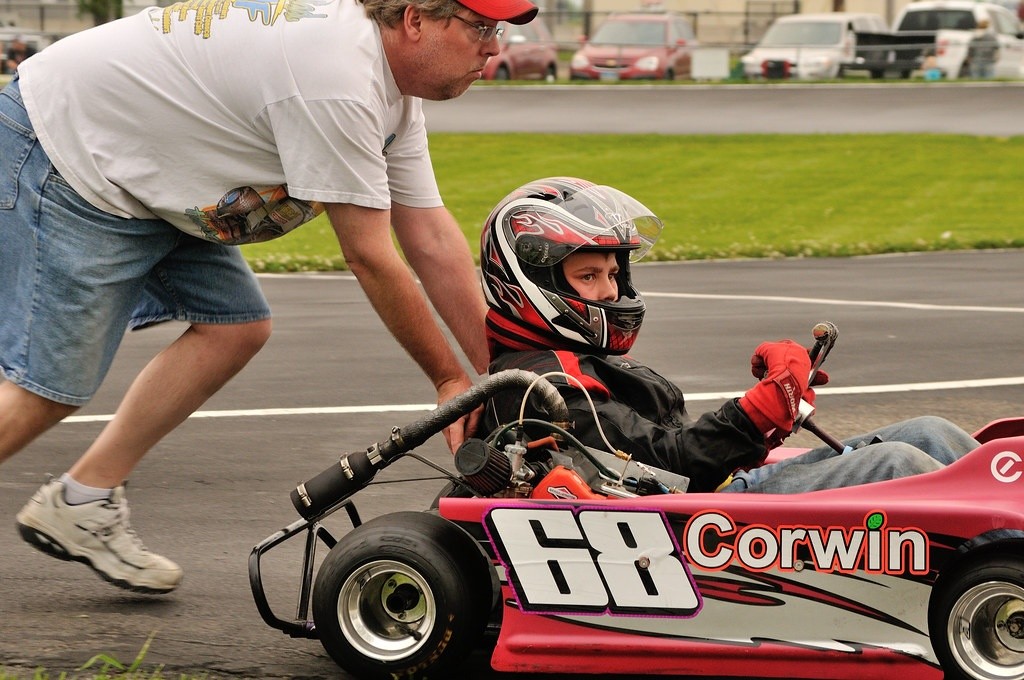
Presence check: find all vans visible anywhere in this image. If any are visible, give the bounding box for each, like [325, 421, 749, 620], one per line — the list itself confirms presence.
[889, 2, 1024, 81]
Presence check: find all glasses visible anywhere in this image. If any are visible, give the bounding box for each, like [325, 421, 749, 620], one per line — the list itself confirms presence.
[422, 3, 505, 43]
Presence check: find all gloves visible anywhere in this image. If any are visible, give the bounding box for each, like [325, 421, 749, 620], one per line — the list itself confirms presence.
[738, 339, 829, 452]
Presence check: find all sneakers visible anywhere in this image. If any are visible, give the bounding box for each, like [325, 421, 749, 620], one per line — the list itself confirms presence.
[15, 473, 185, 595]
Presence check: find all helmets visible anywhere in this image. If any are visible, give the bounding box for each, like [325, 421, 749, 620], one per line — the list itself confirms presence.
[481, 177, 646, 363]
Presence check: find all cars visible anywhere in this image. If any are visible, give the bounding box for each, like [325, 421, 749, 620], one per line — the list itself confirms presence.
[474, 13, 559, 83]
[565, 11, 702, 84]
[736, 12, 885, 85]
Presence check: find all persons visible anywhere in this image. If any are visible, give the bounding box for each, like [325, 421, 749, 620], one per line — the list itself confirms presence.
[0, 0, 538, 595]
[474, 177, 983, 493]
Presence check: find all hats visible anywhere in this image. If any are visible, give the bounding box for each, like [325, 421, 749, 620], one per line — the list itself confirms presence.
[457, 0, 539, 26]
